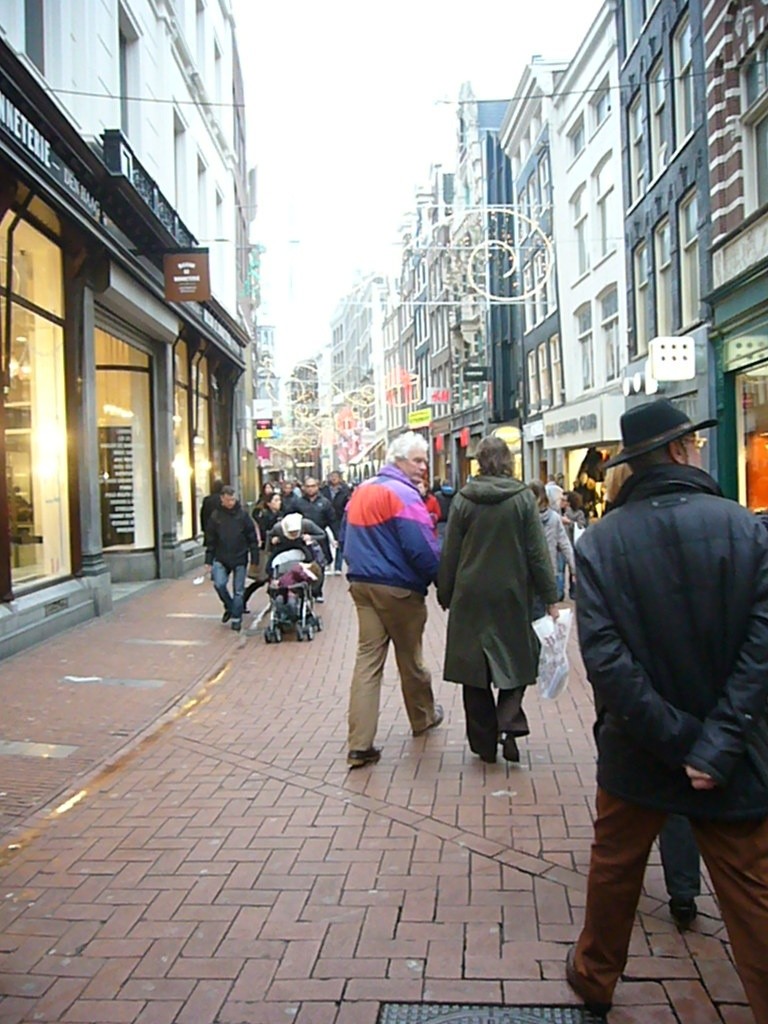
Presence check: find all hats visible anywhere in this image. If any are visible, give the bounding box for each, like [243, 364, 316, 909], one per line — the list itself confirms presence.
[599, 399, 720, 468]
[280, 513, 303, 540]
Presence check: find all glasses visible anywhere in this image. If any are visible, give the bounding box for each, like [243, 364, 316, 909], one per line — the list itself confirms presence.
[678, 433, 707, 448]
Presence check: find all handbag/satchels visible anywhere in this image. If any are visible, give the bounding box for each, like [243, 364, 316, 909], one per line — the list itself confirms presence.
[246, 550, 271, 582]
[532, 607, 573, 698]
[572, 522, 585, 548]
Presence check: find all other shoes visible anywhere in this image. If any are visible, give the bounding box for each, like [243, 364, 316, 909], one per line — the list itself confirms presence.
[222, 610, 231, 623]
[346, 746, 381, 765]
[324, 568, 333, 576]
[231, 619, 240, 631]
[244, 608, 250, 614]
[503, 734, 519, 762]
[412, 705, 444, 735]
[479, 747, 497, 762]
[569, 588, 577, 600]
[334, 570, 341, 575]
[667, 895, 696, 932]
[315, 596, 324, 603]
[566, 942, 614, 1013]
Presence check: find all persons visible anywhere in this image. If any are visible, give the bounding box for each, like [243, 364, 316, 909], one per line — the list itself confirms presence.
[529, 467, 586, 636]
[201, 482, 226, 581]
[263, 511, 329, 604]
[339, 429, 444, 767]
[252, 472, 354, 577]
[565, 393, 768, 1024]
[203, 487, 258, 631]
[418, 477, 459, 543]
[434, 437, 563, 763]
[243, 493, 288, 614]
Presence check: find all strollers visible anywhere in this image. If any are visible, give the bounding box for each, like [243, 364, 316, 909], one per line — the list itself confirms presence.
[264, 539, 323, 644]
